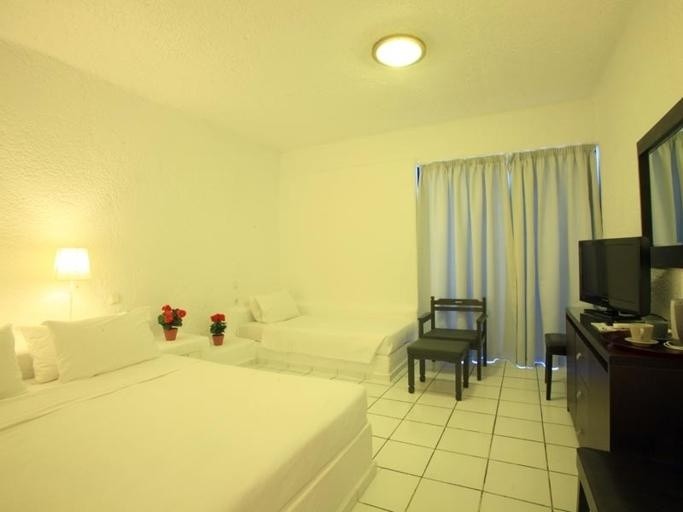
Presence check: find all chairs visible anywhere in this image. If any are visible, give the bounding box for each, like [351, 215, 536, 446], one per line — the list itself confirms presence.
[416, 296, 488, 382]
[570, 447, 683, 512]
[543, 333, 568, 401]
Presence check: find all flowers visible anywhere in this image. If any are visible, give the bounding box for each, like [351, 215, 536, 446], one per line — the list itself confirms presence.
[156, 303, 186, 328]
[208, 314, 226, 335]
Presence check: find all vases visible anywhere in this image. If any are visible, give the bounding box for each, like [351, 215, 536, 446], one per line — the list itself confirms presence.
[163, 328, 178, 341]
[211, 334, 224, 346]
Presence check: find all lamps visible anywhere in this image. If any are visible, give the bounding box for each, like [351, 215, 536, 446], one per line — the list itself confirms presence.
[53, 249, 90, 316]
[372, 34, 426, 69]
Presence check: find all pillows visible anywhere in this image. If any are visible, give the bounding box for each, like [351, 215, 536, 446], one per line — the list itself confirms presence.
[0, 323, 20, 399]
[17, 320, 60, 383]
[251, 290, 298, 322]
[43, 307, 162, 381]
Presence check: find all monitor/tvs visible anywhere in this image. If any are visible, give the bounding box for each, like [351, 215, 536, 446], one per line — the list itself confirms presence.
[578, 237, 651, 319]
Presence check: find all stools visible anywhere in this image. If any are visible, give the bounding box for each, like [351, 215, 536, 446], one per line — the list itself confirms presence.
[407, 338, 470, 400]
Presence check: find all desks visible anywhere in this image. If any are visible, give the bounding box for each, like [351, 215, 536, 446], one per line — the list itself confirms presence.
[564, 306, 683, 452]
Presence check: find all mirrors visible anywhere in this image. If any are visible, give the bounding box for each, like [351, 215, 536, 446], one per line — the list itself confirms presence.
[636, 99, 683, 268]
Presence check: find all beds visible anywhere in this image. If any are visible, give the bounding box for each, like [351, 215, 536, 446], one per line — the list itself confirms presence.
[0, 305, 377, 512]
[237, 290, 416, 386]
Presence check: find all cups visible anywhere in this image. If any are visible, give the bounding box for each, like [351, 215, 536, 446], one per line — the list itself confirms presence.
[630, 323, 654, 341]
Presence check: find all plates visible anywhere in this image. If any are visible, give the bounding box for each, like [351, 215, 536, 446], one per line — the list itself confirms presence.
[626, 337, 658, 346]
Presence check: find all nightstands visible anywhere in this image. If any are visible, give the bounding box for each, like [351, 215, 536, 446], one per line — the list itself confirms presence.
[199, 332, 261, 368]
[156, 333, 202, 357]
